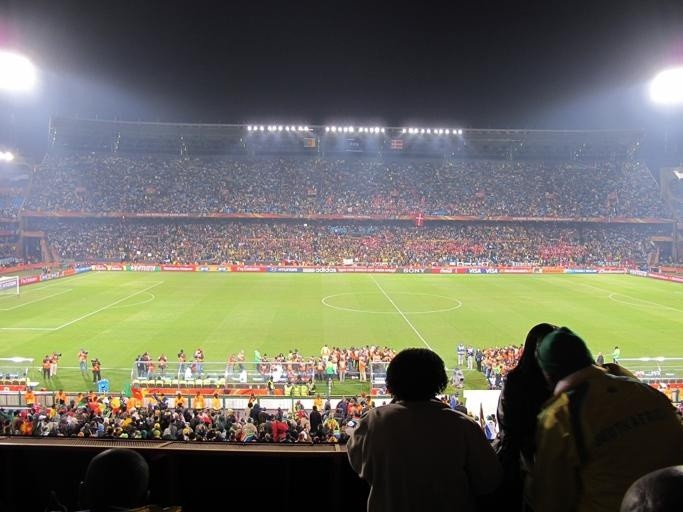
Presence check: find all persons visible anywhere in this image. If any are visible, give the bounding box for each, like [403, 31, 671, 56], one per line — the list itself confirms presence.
[457, 344, 465, 365]
[612, 346, 620, 364]
[194, 349, 204, 372]
[77, 448, 149, 511]
[256, 349, 325, 396]
[78, 349, 89, 376]
[468, 411, 496, 443]
[497, 325, 559, 510]
[228, 350, 246, 372]
[532, 329, 682, 510]
[42, 265, 48, 274]
[441, 391, 466, 414]
[43, 352, 59, 380]
[347, 348, 498, 510]
[476, 346, 523, 390]
[596, 353, 604, 365]
[91, 358, 101, 383]
[178, 349, 186, 372]
[2, 156, 679, 267]
[316, 344, 396, 382]
[451, 367, 465, 388]
[136, 352, 153, 377]
[467, 345, 475, 369]
[1, 388, 375, 446]
[158, 354, 168, 376]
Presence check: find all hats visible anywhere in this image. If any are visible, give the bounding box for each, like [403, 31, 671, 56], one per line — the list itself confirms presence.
[536, 327, 592, 385]
